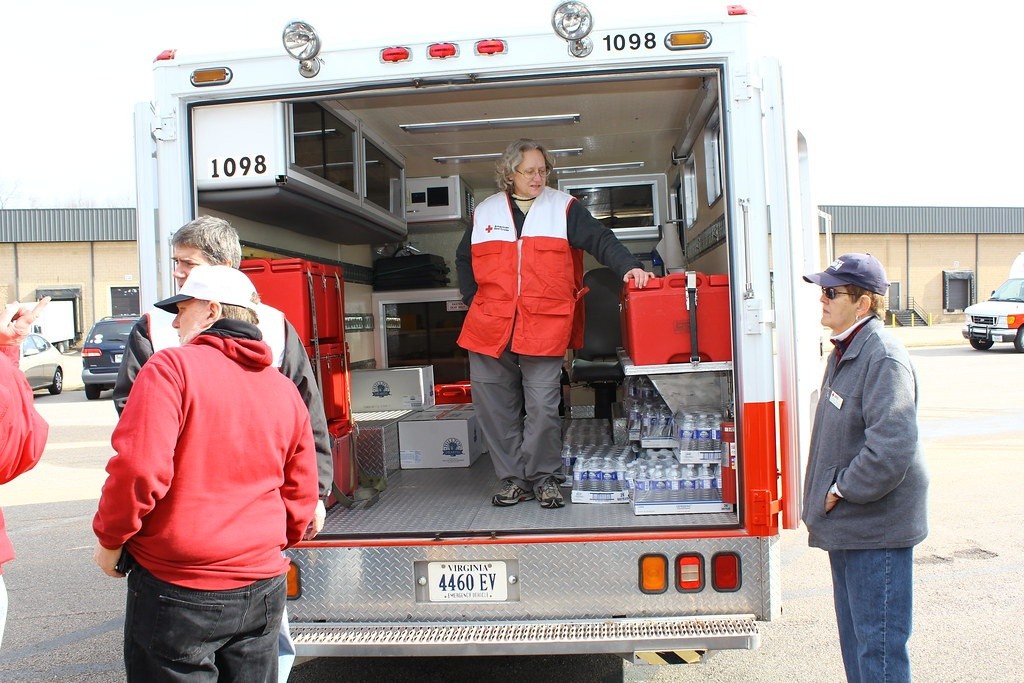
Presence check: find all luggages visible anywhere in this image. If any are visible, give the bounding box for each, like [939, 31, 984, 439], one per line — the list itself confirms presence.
[374, 253, 451, 288]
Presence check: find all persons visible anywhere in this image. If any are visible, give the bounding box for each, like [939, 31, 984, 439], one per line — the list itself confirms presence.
[454, 138, 655, 508]
[0, 294, 53, 649]
[112, 214, 333, 683]
[800, 251, 929, 683]
[92, 263, 318, 683]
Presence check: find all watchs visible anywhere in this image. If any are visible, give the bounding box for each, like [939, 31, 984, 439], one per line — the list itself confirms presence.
[830, 482, 843, 499]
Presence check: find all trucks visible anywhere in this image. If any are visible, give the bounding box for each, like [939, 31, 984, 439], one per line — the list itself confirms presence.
[961, 252, 1024, 355]
[133, 4, 848, 657]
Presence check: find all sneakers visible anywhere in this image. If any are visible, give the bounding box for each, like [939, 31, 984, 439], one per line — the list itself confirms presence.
[493, 480, 535, 505]
[535, 478, 566, 508]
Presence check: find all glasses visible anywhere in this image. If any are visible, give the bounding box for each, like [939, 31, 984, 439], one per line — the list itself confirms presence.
[516, 168, 551, 179]
[821, 285, 856, 299]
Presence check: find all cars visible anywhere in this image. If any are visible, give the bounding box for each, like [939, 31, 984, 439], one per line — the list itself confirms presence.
[19, 332, 65, 395]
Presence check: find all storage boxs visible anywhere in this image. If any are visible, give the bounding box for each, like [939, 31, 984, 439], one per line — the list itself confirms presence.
[350, 365, 435, 412]
[397, 402, 481, 469]
[434, 380, 472, 404]
[619, 271, 731, 367]
[239, 258, 358, 508]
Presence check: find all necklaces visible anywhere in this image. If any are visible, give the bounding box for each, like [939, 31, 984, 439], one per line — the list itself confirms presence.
[511, 196, 536, 201]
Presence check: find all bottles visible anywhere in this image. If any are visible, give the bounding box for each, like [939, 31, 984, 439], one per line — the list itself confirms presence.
[557, 373, 724, 501]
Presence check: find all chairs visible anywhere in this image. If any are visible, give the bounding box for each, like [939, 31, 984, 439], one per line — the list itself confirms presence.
[571, 267, 621, 421]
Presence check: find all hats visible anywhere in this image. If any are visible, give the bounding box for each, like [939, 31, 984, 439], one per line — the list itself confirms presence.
[154, 264, 260, 314]
[802, 253, 890, 296]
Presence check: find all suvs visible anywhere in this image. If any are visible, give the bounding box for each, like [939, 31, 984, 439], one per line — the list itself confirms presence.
[81, 314, 145, 400]
